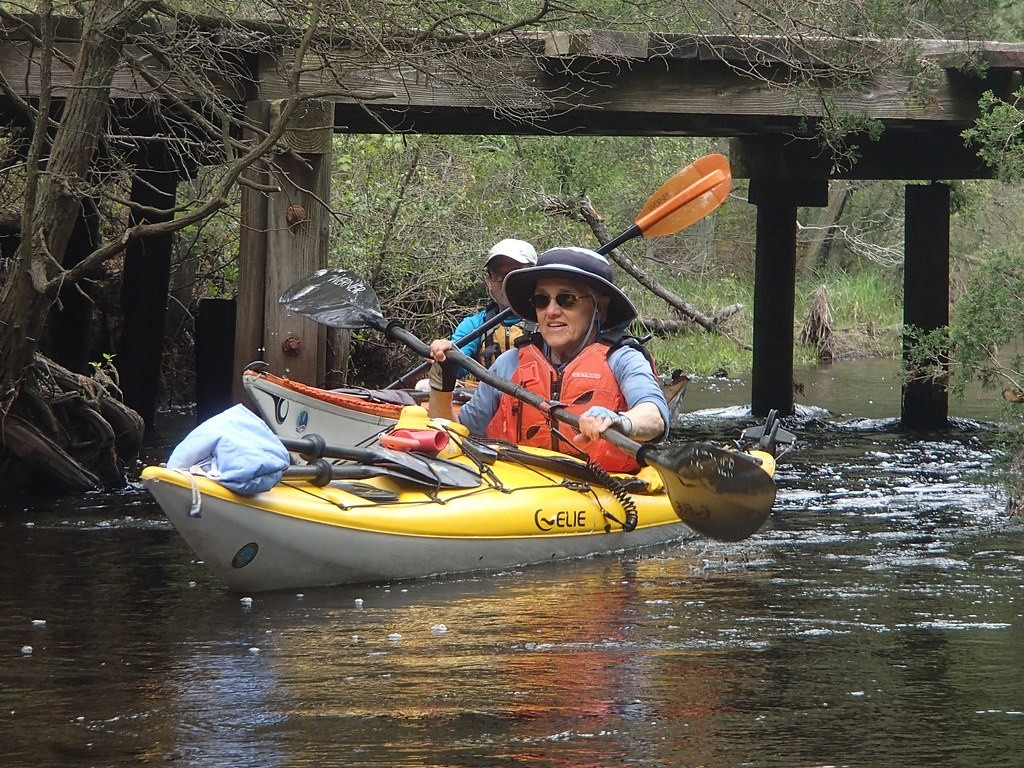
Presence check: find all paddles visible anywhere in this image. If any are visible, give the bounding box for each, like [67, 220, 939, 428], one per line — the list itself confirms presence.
[279, 268, 777, 545]
[382, 152, 732, 388]
[277, 433, 482, 489]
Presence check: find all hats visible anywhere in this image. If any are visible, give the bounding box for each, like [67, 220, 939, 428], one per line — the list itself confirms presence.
[483, 239, 537, 268]
[502, 247, 638, 331]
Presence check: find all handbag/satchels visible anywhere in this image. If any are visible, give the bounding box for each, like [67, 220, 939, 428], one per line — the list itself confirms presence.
[160, 403, 291, 518]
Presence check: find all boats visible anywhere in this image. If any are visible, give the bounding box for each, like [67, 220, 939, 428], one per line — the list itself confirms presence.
[243, 361, 690, 462]
[141, 408, 797, 591]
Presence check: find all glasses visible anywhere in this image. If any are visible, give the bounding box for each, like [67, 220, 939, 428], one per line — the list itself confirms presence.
[529, 293, 592, 308]
[487, 271, 508, 282]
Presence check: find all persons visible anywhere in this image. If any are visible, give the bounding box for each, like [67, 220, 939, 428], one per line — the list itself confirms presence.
[428, 248, 670, 477]
[414, 237, 538, 395]
